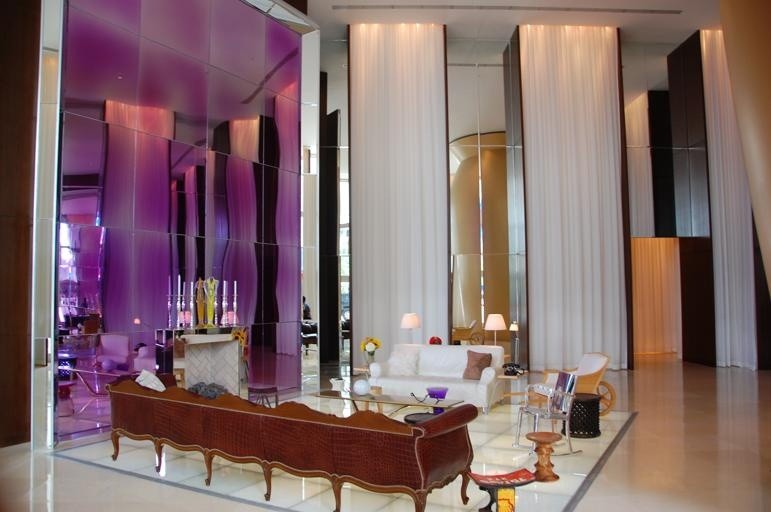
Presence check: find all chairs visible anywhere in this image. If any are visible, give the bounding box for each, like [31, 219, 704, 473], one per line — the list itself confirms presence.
[514, 371, 584, 456]
[452, 320, 484, 345]
[521, 352, 616, 425]
[133, 345, 157, 374]
[97, 334, 130, 365]
[301, 319, 350, 356]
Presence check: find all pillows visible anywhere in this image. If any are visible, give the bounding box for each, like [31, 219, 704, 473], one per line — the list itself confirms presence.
[134, 369, 167, 392]
[388, 352, 419, 376]
[462, 350, 492, 379]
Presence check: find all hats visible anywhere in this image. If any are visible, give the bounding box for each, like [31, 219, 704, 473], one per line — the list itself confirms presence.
[134, 343, 147, 351]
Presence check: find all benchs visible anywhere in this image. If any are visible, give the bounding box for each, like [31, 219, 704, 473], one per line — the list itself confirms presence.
[105, 371, 479, 512]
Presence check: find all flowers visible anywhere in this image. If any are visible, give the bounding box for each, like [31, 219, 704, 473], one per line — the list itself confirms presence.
[360, 336, 381, 354]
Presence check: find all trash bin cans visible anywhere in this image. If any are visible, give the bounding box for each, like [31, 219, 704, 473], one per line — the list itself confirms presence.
[561, 393, 601, 438]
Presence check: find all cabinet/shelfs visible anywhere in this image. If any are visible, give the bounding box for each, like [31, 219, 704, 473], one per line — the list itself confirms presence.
[55, 305, 101, 395]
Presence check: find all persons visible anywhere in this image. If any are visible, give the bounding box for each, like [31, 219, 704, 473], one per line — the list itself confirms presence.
[303, 295, 311, 355]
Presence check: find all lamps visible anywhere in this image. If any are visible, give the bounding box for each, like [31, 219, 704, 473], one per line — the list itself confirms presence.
[509, 320, 520, 338]
[400, 313, 421, 344]
[485, 312, 507, 346]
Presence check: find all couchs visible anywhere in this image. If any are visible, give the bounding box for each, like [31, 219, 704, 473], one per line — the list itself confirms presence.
[368, 343, 505, 414]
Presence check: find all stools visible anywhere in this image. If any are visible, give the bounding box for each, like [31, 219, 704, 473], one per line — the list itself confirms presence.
[248, 384, 279, 409]
[466, 468, 535, 512]
[526, 433, 564, 482]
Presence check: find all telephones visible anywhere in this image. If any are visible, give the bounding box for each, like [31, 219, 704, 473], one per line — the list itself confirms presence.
[502, 363, 520, 376]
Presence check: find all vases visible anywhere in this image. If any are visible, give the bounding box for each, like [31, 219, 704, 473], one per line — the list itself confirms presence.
[366, 351, 376, 375]
